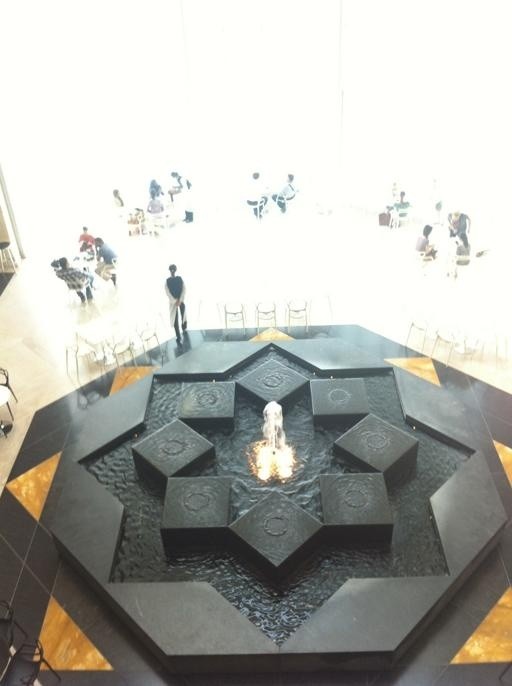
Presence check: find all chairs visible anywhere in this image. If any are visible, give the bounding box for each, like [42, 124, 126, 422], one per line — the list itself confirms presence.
[63, 328, 164, 381]
[405, 322, 507, 368]
[224, 299, 310, 337]
[70, 189, 484, 307]
[1, 600, 61, 686]
[0, 368, 18, 420]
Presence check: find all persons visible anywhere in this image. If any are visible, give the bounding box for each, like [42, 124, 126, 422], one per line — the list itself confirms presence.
[393, 191, 410, 228]
[245, 172, 268, 219]
[414, 225, 436, 262]
[113, 190, 127, 213]
[92, 237, 117, 286]
[151, 180, 163, 195]
[448, 212, 471, 249]
[80, 226, 96, 254]
[56, 257, 94, 305]
[164, 264, 187, 343]
[272, 174, 297, 214]
[146, 188, 165, 216]
[169, 171, 195, 223]
